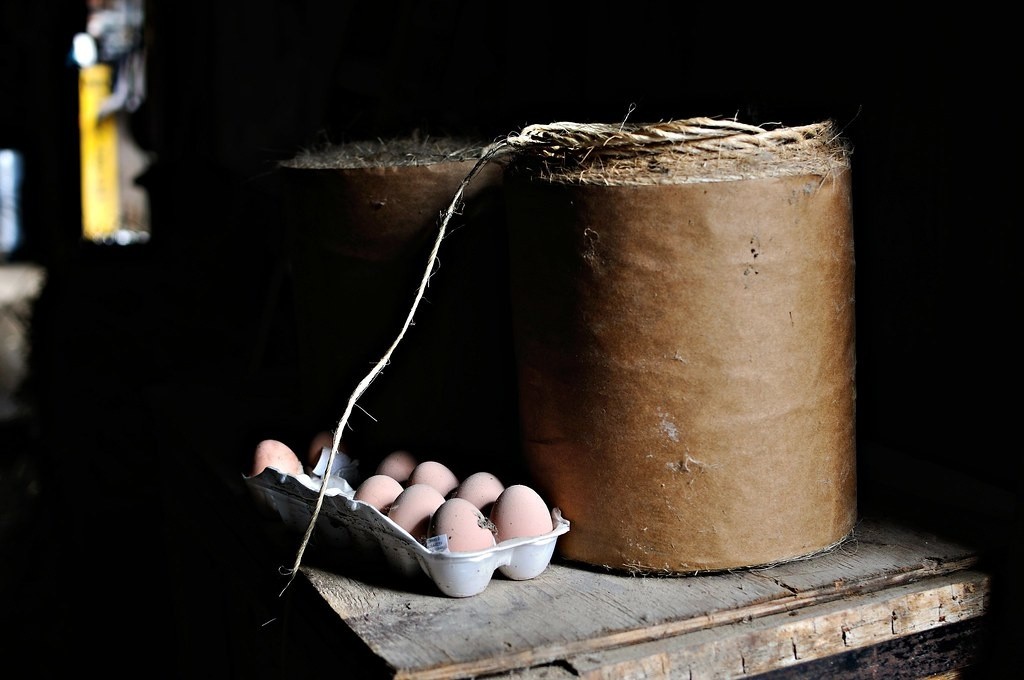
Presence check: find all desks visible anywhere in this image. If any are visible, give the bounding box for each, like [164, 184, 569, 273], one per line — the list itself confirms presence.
[290, 528, 993, 680]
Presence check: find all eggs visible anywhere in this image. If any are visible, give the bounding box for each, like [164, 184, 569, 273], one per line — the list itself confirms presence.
[248, 426, 553, 553]
